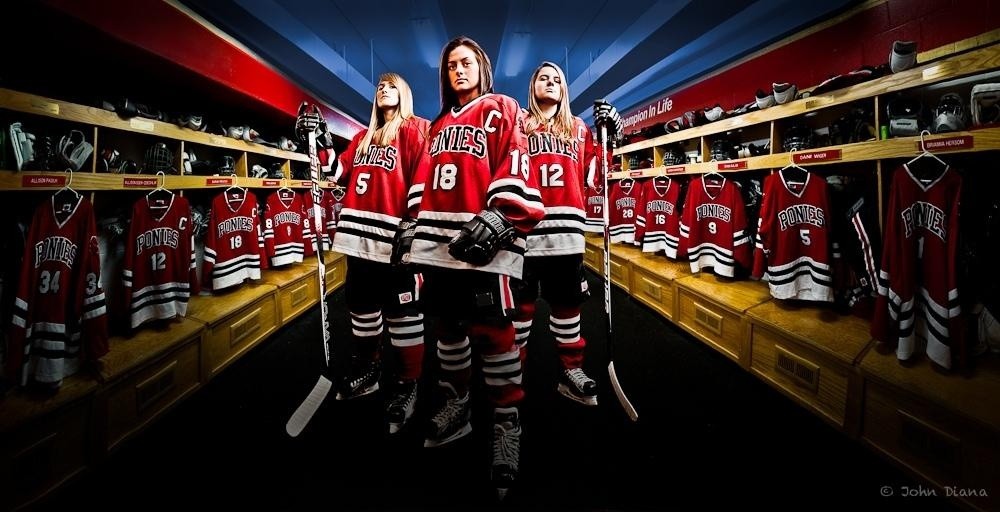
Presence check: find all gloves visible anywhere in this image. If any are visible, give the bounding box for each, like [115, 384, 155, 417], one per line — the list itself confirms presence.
[593, 97, 624, 150]
[446, 208, 519, 269]
[293, 101, 339, 150]
[389, 215, 419, 269]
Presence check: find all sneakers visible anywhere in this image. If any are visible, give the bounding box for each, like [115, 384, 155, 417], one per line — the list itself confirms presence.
[423, 380, 472, 441]
[337, 354, 383, 395]
[383, 379, 420, 424]
[489, 404, 523, 489]
[558, 367, 599, 396]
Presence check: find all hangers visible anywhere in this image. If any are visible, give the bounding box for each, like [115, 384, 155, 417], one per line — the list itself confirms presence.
[145, 170, 172, 210]
[50, 166, 82, 215]
[898, 125, 946, 184]
[773, 140, 814, 189]
[223, 171, 246, 203]
[652, 163, 671, 190]
[702, 157, 725, 190]
[317, 186, 324, 198]
[620, 166, 635, 190]
[276, 175, 295, 204]
[332, 185, 344, 198]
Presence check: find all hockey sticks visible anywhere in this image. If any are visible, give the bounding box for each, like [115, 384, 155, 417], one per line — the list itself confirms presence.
[286, 131, 333, 437]
[601, 124, 639, 423]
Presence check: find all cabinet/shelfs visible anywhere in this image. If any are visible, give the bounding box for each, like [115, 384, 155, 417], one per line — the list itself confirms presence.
[0, 82, 350, 512]
[581, 40, 1000, 511]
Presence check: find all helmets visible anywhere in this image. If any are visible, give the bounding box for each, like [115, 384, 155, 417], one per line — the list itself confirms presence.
[662, 147, 686, 166]
[217, 155, 235, 178]
[709, 137, 734, 161]
[138, 139, 176, 176]
[781, 119, 817, 155]
[628, 152, 647, 171]
[930, 91, 971, 133]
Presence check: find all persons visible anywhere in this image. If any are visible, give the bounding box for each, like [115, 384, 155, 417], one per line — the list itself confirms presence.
[294, 71, 432, 421]
[407, 36, 544, 491]
[507, 61, 621, 398]
